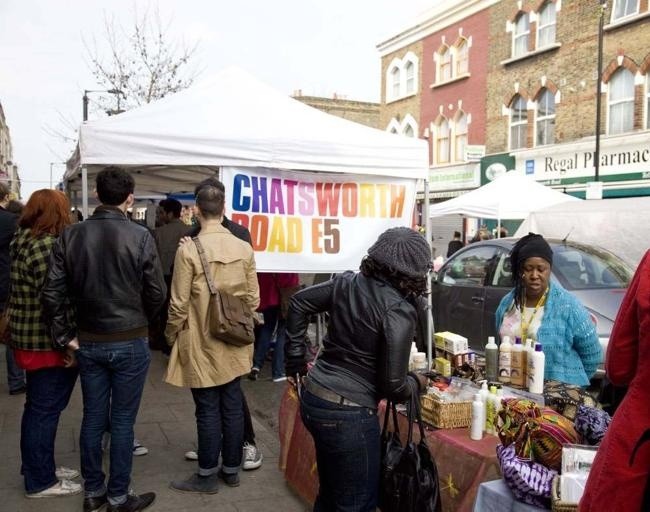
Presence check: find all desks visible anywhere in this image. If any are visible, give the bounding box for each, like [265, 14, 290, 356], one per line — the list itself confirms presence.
[474, 477, 551, 512]
[279, 363, 503, 512]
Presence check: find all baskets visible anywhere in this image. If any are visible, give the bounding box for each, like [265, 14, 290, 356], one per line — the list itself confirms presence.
[552, 473, 578, 512]
[417, 394, 472, 430]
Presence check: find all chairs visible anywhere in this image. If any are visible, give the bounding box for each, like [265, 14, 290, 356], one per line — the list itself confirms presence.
[498, 257, 513, 287]
[559, 262, 586, 285]
[455, 264, 484, 288]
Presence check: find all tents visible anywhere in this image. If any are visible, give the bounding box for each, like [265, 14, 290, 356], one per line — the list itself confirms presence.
[60, 67, 433, 386]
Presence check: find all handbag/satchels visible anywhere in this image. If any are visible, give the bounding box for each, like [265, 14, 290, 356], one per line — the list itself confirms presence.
[495, 445, 559, 509]
[208, 287, 256, 347]
[544, 379, 611, 447]
[380, 428, 443, 511]
[1, 314, 11, 346]
[500, 396, 589, 472]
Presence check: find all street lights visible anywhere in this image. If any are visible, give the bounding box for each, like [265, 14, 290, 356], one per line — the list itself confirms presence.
[82, 87, 125, 121]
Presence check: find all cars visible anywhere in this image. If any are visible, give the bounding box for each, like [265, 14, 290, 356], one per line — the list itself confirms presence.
[413, 235, 637, 381]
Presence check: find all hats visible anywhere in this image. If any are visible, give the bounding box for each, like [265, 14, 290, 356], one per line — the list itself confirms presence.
[367, 226, 431, 277]
[515, 239, 552, 264]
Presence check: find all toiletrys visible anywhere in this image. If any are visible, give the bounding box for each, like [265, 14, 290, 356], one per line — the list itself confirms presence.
[511, 337, 524, 385]
[485, 386, 497, 434]
[528, 344, 545, 393]
[478, 380, 489, 400]
[524, 340, 536, 387]
[485, 335, 498, 380]
[471, 392, 484, 442]
[498, 336, 512, 382]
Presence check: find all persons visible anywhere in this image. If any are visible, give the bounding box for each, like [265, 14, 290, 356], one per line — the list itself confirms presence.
[4, 189, 83, 500]
[37, 166, 167, 511]
[494, 222, 506, 238]
[282, 225, 435, 512]
[575, 245, 649, 512]
[169, 177, 264, 471]
[249, 273, 300, 383]
[446, 231, 464, 259]
[149, 199, 190, 353]
[493, 231, 603, 393]
[160, 186, 261, 495]
[1, 177, 82, 394]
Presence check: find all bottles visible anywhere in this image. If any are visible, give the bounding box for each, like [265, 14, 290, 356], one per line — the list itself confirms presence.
[408, 341, 428, 373]
[470, 335, 545, 441]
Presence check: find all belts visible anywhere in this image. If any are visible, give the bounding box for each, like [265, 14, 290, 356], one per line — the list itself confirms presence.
[303, 371, 362, 408]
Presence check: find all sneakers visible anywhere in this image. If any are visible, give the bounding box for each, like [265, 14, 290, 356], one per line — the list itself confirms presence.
[185, 449, 200, 461]
[169, 473, 218, 493]
[132, 437, 148, 455]
[271, 373, 288, 383]
[218, 465, 240, 486]
[10, 381, 26, 395]
[248, 366, 260, 382]
[243, 444, 263, 472]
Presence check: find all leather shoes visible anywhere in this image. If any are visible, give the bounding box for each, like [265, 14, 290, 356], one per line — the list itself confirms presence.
[55, 466, 80, 480]
[26, 480, 83, 498]
[83, 490, 156, 511]
